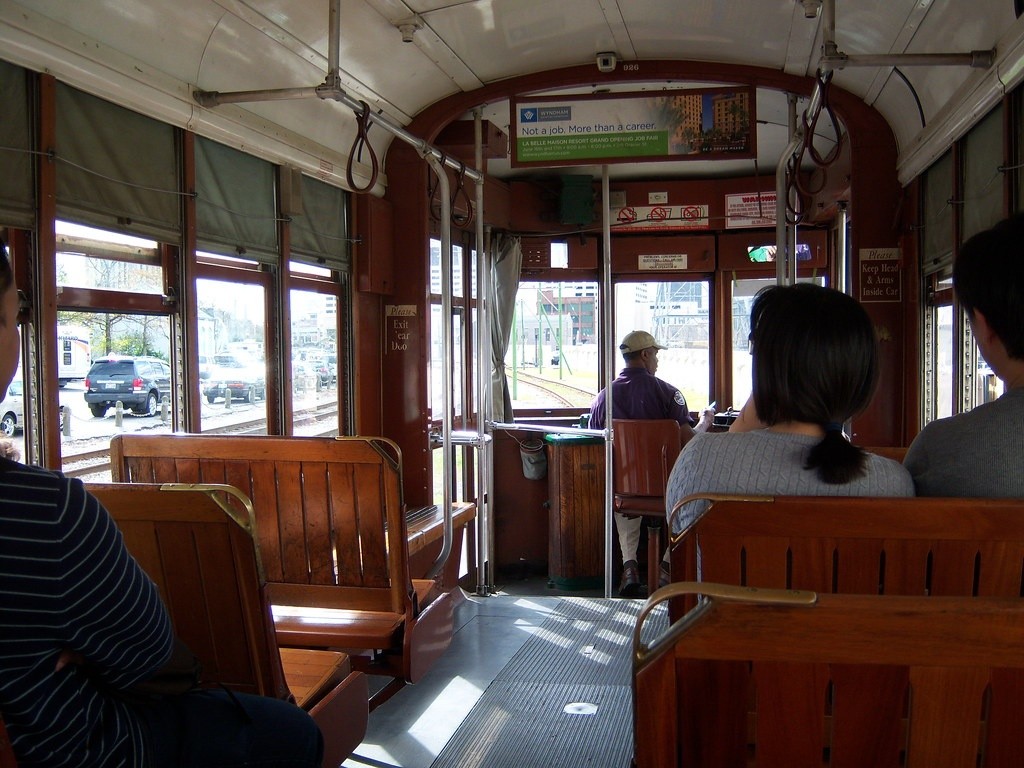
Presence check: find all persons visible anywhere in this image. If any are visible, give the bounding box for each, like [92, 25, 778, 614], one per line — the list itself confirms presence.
[591, 331, 717, 595]
[666, 282, 914, 536]
[0, 246, 327, 767]
[904, 216, 1023, 501]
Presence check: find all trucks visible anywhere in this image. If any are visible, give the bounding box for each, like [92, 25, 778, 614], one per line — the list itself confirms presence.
[12, 326, 92, 391]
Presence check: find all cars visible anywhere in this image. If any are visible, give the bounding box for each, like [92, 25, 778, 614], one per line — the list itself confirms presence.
[0, 378, 65, 438]
[199, 340, 338, 405]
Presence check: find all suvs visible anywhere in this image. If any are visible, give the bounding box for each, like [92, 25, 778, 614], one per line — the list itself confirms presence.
[84, 355, 170, 418]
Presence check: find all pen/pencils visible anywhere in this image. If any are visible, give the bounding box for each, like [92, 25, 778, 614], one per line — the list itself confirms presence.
[707, 400, 717, 409]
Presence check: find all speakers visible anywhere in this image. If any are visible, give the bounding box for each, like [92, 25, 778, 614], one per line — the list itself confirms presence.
[538, 174, 594, 225]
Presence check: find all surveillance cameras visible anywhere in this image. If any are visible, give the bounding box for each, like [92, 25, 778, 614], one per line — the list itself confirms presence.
[596, 55, 616, 73]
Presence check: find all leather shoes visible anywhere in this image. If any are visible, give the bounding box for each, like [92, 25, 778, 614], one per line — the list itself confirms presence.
[657, 565, 671, 588]
[617, 566, 643, 598]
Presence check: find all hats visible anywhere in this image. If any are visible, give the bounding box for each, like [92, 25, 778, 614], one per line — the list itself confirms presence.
[620, 330, 668, 355]
